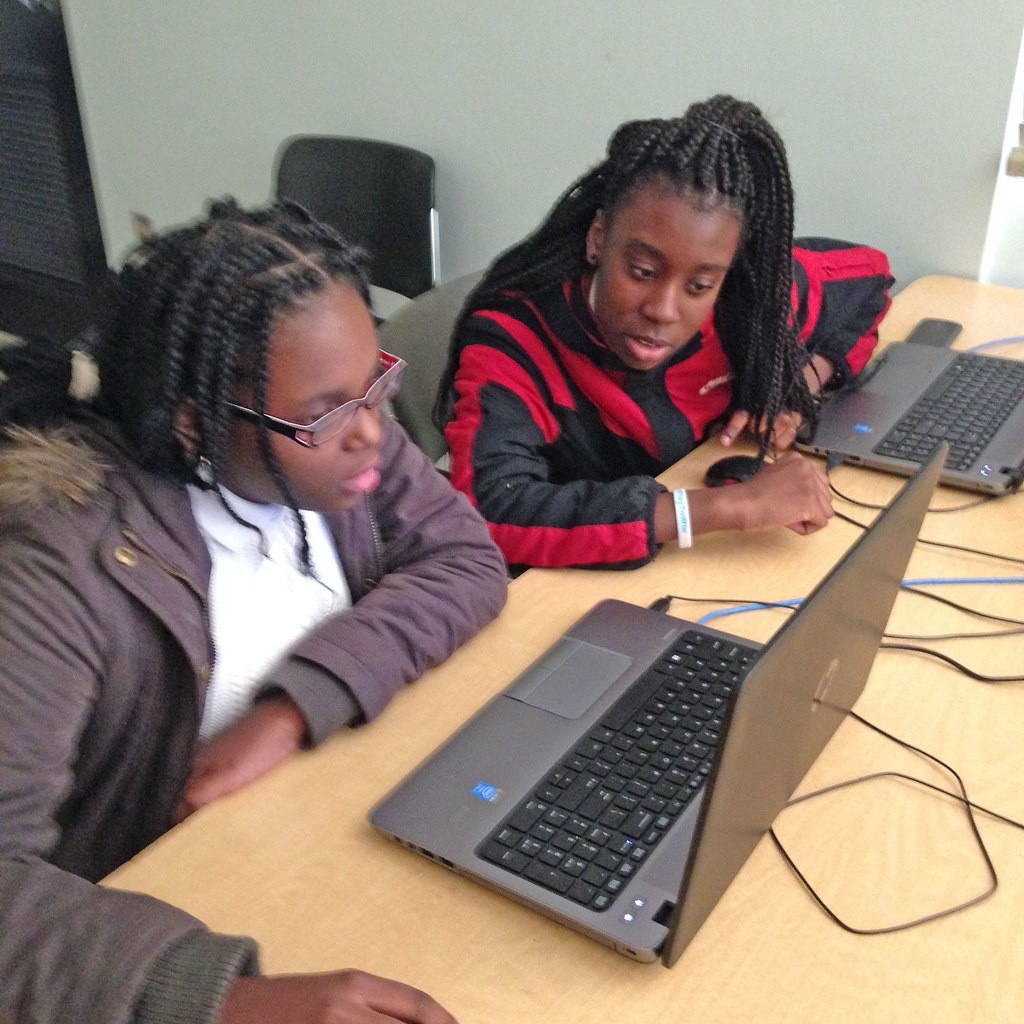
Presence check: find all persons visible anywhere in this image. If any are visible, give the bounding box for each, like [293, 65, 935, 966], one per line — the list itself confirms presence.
[0, 193, 507, 1023]
[430, 94, 895, 581]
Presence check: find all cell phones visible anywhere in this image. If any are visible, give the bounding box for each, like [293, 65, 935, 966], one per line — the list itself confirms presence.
[905, 318, 962, 348]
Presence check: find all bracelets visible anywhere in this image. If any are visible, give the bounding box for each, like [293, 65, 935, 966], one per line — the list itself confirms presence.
[673, 489, 692, 548]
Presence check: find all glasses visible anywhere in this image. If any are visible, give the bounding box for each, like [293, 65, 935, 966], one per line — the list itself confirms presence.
[222, 348, 406, 448]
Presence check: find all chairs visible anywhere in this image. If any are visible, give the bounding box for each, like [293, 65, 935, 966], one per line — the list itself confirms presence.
[273, 133, 448, 331]
[0, 260, 120, 441]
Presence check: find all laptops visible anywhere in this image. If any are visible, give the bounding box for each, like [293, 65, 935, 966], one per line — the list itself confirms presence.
[368, 442, 949, 969]
[796, 341, 1024, 498]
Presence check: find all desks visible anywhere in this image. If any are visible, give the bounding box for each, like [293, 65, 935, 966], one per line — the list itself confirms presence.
[97, 269, 1024, 1024]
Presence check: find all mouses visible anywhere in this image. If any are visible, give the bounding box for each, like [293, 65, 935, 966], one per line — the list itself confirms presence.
[703, 454, 771, 489]
